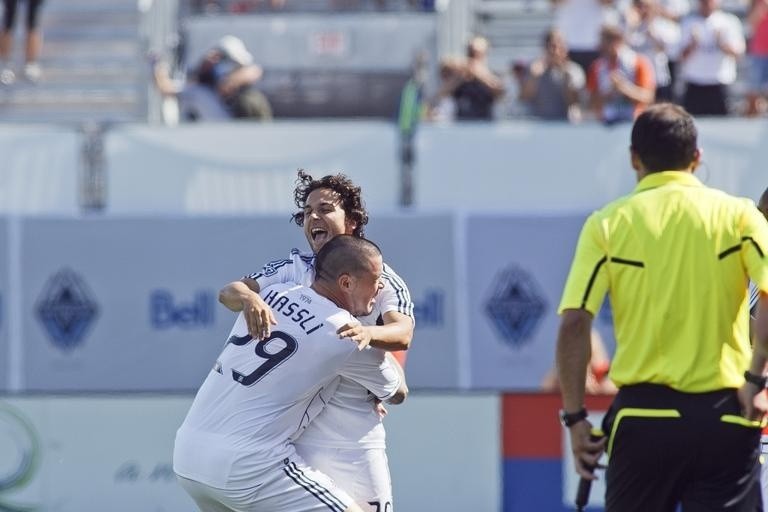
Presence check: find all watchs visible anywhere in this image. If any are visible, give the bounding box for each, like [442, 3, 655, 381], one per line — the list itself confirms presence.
[558, 408, 588, 428]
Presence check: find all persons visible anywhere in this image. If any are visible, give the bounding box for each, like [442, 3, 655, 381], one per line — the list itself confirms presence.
[185, 37, 275, 125]
[170, 232, 411, 512]
[556, 0, 767, 124]
[2, 2, 49, 85]
[540, 327, 621, 397]
[744, 186, 768, 512]
[219, 167, 418, 511]
[553, 102, 768, 511]
[413, 26, 656, 125]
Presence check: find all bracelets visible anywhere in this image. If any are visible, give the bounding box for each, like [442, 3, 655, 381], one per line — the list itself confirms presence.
[746, 370, 768, 391]
